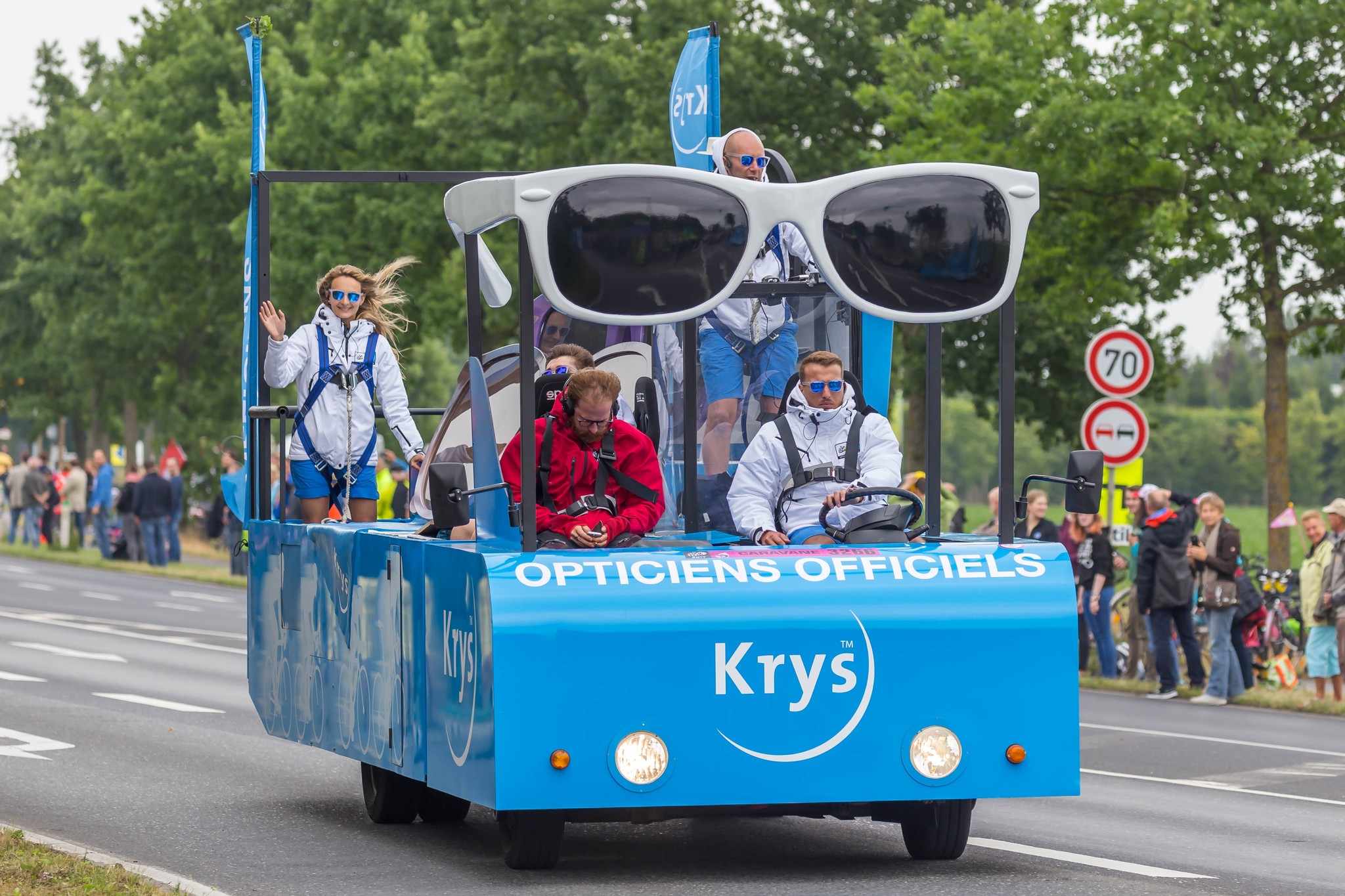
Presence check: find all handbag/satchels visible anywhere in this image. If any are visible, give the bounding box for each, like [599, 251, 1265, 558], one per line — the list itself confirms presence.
[1202, 580, 1237, 611]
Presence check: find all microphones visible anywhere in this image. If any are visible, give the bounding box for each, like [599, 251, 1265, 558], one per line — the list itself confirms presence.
[810, 414, 820, 425]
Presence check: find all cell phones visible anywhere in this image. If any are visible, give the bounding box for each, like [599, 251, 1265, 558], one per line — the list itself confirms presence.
[587, 531, 602, 538]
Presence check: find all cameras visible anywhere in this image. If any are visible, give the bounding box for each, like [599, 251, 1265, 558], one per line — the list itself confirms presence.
[1190, 535, 1200, 547]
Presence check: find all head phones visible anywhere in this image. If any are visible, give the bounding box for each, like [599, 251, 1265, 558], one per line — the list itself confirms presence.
[560, 371, 621, 416]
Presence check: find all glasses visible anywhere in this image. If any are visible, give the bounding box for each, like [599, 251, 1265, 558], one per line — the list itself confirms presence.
[545, 325, 570, 337]
[328, 288, 363, 302]
[574, 405, 614, 427]
[723, 152, 771, 168]
[540, 366, 578, 376]
[801, 380, 844, 393]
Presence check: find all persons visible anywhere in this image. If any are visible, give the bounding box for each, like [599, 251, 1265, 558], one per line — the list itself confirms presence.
[725, 352, 925, 545]
[1137, 488, 1207, 699]
[259, 256, 425, 524]
[894, 471, 1345, 705]
[498, 367, 665, 548]
[702, 128, 820, 536]
[1, 448, 410, 575]
[539, 309, 572, 361]
[545, 343, 637, 429]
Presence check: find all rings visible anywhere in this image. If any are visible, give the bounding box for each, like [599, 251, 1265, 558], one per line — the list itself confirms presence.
[594, 542, 597, 547]
[575, 534, 579, 540]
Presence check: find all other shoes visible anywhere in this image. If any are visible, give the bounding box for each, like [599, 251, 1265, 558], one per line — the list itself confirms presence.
[1146, 687, 1178, 699]
[1191, 694, 1227, 706]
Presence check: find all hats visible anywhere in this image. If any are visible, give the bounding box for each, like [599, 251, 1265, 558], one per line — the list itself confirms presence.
[1193, 492, 1214, 506]
[1133, 483, 1159, 502]
[1322, 498, 1345, 518]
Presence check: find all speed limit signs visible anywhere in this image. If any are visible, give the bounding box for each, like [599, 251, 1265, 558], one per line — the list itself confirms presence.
[1083, 325, 1154, 399]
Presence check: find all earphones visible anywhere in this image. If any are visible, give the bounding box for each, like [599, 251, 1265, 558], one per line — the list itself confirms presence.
[326, 295, 330, 300]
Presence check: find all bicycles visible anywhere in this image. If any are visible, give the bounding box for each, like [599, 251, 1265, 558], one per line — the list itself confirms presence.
[1246, 555, 1304, 666]
